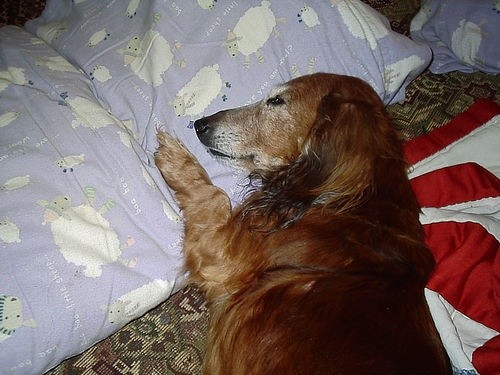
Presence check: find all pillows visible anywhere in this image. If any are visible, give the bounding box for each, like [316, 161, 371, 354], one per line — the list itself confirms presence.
[0, 0, 500, 375]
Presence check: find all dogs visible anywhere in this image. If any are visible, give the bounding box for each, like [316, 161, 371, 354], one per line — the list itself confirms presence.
[154, 72, 454, 375]
